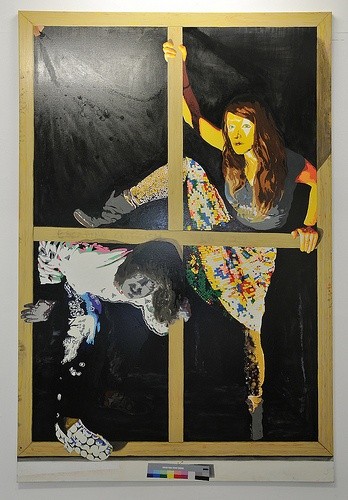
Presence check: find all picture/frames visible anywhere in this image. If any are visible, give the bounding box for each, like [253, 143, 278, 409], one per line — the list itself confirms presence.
[15, 9, 336, 463]
